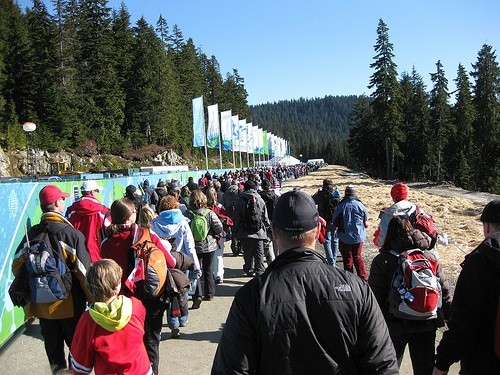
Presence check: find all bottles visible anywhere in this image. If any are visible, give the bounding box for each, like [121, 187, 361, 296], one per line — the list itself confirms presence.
[404, 291, 414, 302]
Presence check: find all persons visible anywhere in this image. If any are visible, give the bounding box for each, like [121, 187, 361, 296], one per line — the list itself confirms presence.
[373, 183, 448, 248]
[211, 188, 399, 375]
[310, 179, 342, 267]
[12, 162, 323, 375]
[68, 258, 155, 375]
[433, 198, 500, 375]
[332, 185, 368, 281]
[367, 216, 454, 375]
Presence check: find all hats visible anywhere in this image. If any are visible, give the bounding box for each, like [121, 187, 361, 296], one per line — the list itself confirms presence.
[345, 184, 356, 195]
[80, 180, 101, 191]
[391, 182, 407, 200]
[479, 200, 500, 222]
[39, 185, 70, 204]
[274, 186, 321, 232]
[143, 179, 149, 186]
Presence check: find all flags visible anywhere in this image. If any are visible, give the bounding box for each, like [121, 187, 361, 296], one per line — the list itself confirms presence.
[207, 104, 219, 148]
[191, 96, 206, 147]
[220, 109, 232, 150]
[231, 115, 290, 158]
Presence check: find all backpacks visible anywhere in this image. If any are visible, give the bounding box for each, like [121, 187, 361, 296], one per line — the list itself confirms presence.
[387, 247, 444, 322]
[187, 209, 212, 242]
[240, 197, 263, 235]
[19, 228, 71, 305]
[396, 201, 438, 250]
[322, 191, 342, 224]
[128, 227, 171, 297]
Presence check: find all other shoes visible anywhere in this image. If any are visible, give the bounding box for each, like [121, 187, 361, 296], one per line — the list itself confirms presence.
[172, 329, 180, 338]
[192, 297, 202, 309]
